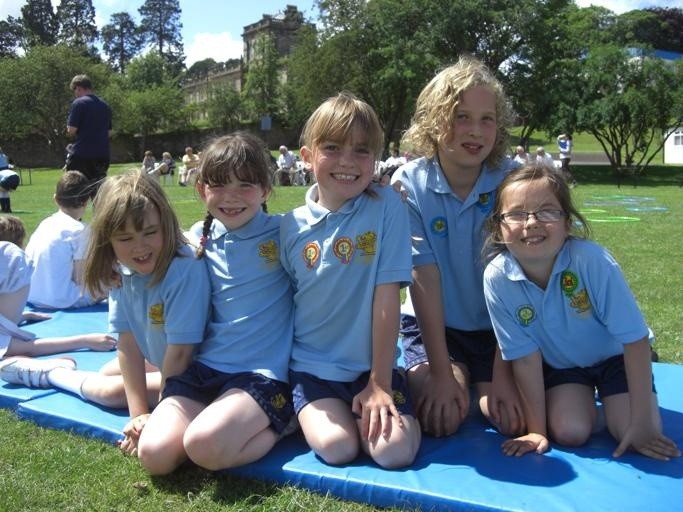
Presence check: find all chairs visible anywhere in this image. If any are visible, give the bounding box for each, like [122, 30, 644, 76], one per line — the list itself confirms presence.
[153, 161, 305, 185]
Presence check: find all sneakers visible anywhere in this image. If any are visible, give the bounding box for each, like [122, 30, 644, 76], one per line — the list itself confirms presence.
[1, 356, 76, 390]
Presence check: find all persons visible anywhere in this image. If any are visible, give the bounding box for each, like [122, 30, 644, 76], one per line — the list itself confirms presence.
[278, 90, 421, 469]
[483, 163, 681, 463]
[0, 241, 118, 357]
[264, 145, 312, 186]
[380, 142, 407, 184]
[0, 168, 20, 213]
[0, 166, 211, 459]
[0, 147, 9, 170]
[389, 55, 528, 440]
[105, 132, 408, 477]
[142, 146, 200, 186]
[63, 73, 113, 222]
[0, 215, 27, 251]
[513, 132, 574, 181]
[23, 168, 123, 310]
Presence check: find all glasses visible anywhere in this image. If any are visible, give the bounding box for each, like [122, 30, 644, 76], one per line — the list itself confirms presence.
[499, 208, 567, 224]
